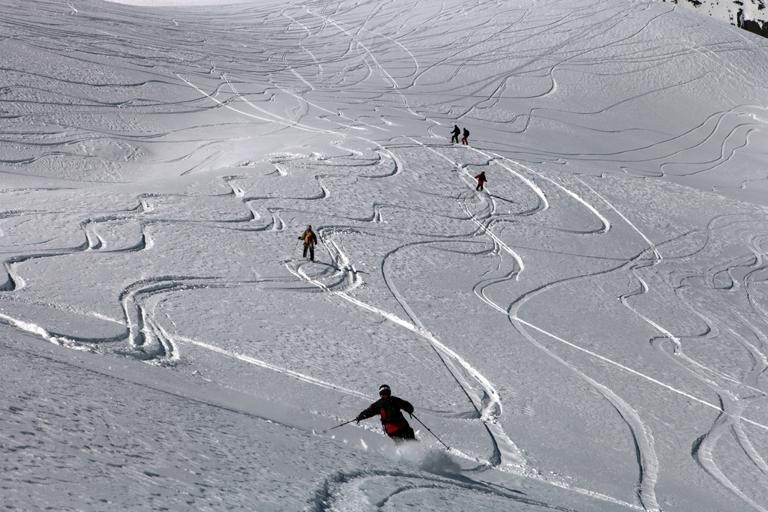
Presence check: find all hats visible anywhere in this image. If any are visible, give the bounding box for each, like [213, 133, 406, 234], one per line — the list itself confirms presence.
[379, 385, 391, 395]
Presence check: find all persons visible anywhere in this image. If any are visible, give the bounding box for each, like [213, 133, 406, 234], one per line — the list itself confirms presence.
[356, 383, 417, 443]
[462, 127, 469, 145]
[450, 125, 460, 144]
[298, 224, 318, 261]
[474, 171, 487, 191]
